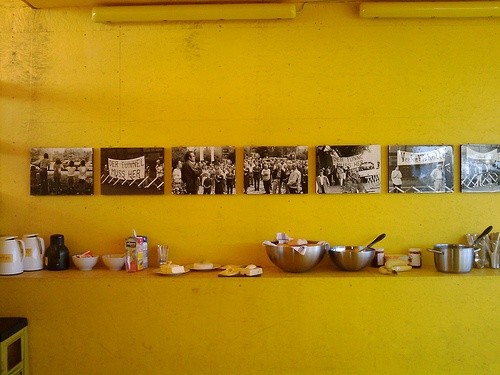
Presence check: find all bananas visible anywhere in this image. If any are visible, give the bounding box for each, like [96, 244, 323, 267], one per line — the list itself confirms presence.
[379, 259, 412, 275]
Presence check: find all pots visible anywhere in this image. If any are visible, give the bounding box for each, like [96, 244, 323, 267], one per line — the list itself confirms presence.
[427, 243, 481, 273]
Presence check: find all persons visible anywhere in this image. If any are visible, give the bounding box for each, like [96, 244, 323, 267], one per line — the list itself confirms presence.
[430, 163, 453, 192]
[77, 160, 88, 194]
[66, 161, 76, 191]
[243, 154, 308, 194]
[462, 159, 500, 187]
[30, 153, 50, 194]
[145, 157, 164, 185]
[53, 158, 64, 191]
[172, 151, 236, 194]
[317, 161, 380, 194]
[391, 166, 402, 193]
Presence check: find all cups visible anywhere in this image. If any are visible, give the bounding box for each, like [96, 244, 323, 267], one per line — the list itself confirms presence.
[156, 243, 168, 267]
[484, 239, 500, 269]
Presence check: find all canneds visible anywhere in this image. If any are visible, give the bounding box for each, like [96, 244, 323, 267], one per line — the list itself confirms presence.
[408, 248, 422, 268]
[371, 248, 385, 267]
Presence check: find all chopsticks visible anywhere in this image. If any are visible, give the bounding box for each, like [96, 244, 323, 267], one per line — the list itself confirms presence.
[485, 231, 499, 268]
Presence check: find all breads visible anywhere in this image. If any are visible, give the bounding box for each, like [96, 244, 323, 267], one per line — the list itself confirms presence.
[160, 264, 185, 273]
[193, 262, 214, 269]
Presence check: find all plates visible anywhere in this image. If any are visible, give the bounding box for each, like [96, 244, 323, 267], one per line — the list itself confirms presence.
[185, 263, 220, 270]
[152, 268, 190, 275]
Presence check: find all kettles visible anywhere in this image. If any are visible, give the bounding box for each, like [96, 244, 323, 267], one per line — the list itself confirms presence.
[0, 235, 26, 276]
[21, 233, 46, 271]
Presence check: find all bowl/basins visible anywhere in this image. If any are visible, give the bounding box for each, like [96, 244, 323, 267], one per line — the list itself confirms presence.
[72, 254, 98, 271]
[265, 240, 325, 273]
[101, 253, 126, 271]
[328, 245, 376, 272]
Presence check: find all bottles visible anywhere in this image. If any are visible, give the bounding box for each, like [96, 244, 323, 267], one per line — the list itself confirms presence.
[44, 234, 69, 270]
